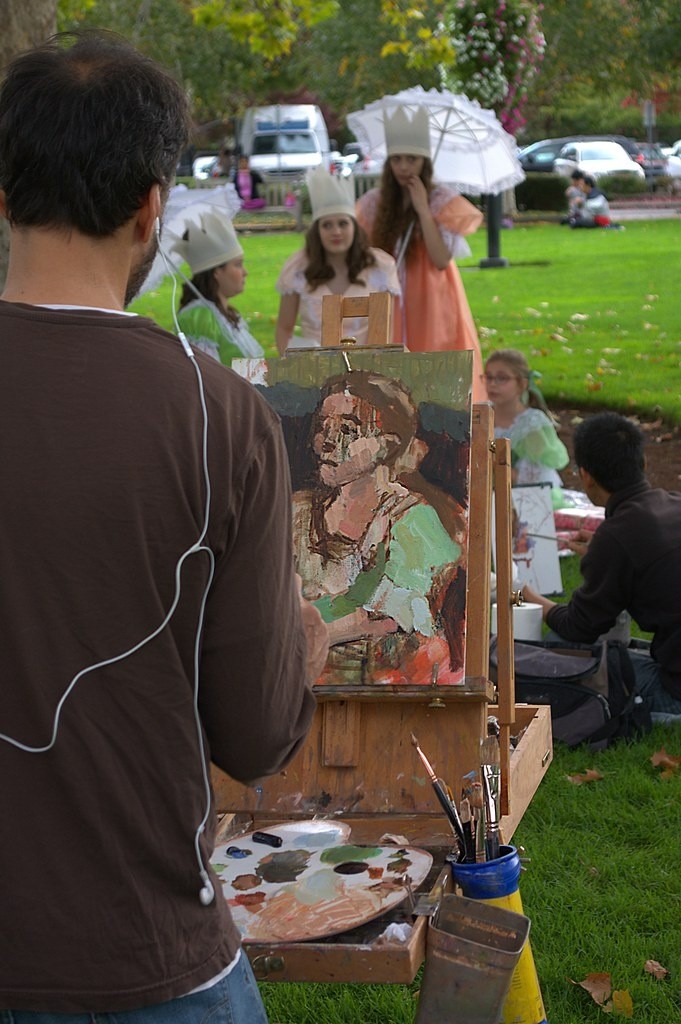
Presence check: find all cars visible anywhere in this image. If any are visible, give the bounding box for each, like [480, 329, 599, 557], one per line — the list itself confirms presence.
[634, 141, 681, 178]
[553, 140, 645, 181]
[518, 134, 645, 172]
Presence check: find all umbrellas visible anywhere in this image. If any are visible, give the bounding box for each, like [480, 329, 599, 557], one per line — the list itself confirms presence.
[345, 84, 528, 271]
[138, 183, 257, 361]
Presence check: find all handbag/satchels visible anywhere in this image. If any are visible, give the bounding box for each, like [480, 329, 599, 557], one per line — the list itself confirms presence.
[487, 634, 652, 751]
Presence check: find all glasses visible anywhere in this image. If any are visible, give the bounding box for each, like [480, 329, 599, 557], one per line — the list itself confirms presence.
[480, 374, 519, 384]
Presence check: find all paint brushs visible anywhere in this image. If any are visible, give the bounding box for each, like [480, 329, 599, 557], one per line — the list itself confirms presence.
[406, 730, 467, 860]
[526, 532, 567, 553]
[440, 765, 500, 862]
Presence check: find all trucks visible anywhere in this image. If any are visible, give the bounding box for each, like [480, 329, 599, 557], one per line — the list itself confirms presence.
[240, 104, 330, 184]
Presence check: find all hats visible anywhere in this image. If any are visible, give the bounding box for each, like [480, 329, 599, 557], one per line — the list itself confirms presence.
[165, 205, 244, 274]
[306, 165, 356, 221]
[384, 105, 431, 159]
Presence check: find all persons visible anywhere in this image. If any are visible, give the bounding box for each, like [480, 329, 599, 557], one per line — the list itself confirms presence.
[271, 201, 403, 356]
[559, 169, 615, 229]
[176, 207, 265, 369]
[520, 413, 681, 716]
[210, 147, 268, 209]
[0, 27, 331, 1024]
[480, 351, 575, 509]
[356, 105, 489, 405]
